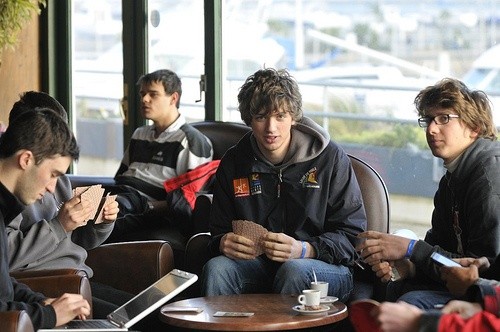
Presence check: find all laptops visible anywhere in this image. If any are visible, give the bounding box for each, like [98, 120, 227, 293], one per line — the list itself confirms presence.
[63, 267, 198, 329]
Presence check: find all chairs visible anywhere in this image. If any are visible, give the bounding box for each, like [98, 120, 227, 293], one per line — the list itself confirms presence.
[0, 121, 391, 332]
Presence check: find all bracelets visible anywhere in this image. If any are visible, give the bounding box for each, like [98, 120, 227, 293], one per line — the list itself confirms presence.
[407, 239, 416, 261]
[300, 241, 306, 261]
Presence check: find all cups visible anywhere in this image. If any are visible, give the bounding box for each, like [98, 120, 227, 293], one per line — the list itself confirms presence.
[311, 281, 329, 298]
[297, 289, 320, 306]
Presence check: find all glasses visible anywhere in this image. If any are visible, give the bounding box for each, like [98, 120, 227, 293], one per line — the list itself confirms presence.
[417, 113, 459, 127]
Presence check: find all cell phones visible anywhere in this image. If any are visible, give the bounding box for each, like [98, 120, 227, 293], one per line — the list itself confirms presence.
[431, 252, 462, 268]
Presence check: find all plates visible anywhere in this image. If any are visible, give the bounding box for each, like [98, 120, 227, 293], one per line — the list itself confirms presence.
[292, 304, 330, 315]
[320, 295, 339, 304]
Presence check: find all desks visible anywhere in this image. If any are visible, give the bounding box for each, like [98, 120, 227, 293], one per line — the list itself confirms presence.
[161, 293, 348, 332]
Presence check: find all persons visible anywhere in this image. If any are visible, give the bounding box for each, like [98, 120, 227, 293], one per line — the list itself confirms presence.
[5, 90, 146, 332]
[198, 68, 361, 301]
[0, 109, 92, 332]
[102, 69, 214, 243]
[355, 77, 500, 332]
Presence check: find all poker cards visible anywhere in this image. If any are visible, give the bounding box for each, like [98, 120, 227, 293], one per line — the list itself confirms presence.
[232, 220, 277, 257]
[72, 184, 118, 224]
[213, 311, 254, 317]
[162, 306, 204, 313]
